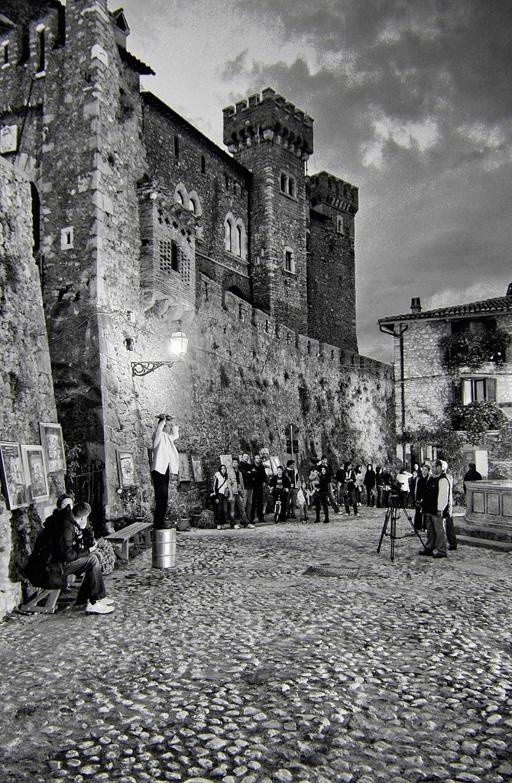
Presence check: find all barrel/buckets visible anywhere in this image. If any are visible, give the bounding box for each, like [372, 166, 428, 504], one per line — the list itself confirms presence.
[151, 528, 177, 569]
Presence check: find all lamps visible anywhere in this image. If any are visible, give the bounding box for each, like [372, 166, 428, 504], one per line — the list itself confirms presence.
[131, 331, 188, 377]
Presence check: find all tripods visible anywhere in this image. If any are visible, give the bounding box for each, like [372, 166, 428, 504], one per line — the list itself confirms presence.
[377, 489, 427, 562]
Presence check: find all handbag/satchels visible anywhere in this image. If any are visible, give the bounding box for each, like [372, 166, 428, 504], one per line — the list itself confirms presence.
[25, 551, 63, 589]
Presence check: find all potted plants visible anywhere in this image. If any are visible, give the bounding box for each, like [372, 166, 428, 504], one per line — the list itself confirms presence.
[130, 506, 215, 531]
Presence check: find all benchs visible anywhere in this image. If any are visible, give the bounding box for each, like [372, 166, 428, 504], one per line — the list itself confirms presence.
[20, 571, 87, 614]
[104, 522, 154, 564]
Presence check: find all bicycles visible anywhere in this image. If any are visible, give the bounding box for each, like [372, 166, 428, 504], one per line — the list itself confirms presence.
[269, 488, 287, 525]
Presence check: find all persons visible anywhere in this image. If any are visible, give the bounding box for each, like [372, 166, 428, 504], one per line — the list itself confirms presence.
[150, 413, 182, 528]
[463, 463, 482, 495]
[211, 452, 458, 558]
[25, 493, 116, 614]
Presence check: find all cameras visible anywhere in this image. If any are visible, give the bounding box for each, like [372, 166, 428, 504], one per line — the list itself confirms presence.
[390, 479, 404, 489]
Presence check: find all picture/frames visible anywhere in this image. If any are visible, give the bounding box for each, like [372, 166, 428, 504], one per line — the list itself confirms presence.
[0, 422, 284, 510]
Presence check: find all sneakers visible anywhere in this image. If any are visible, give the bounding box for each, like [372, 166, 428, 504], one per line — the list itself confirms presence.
[86, 603, 115, 614]
[87, 597, 114, 604]
